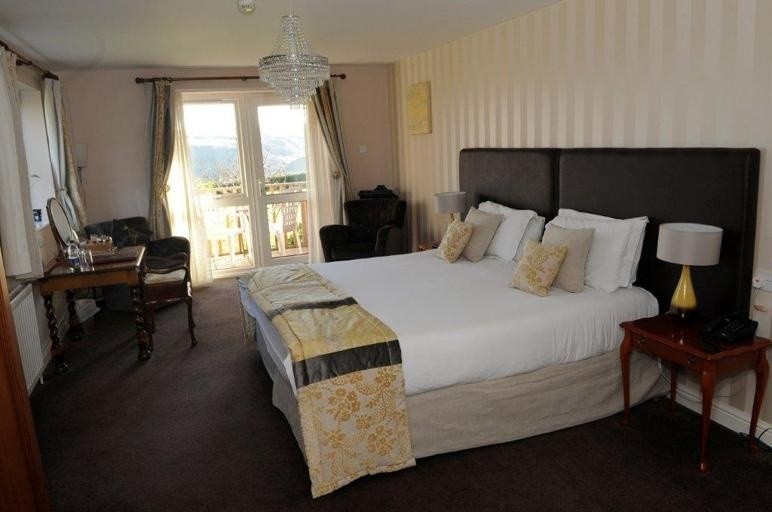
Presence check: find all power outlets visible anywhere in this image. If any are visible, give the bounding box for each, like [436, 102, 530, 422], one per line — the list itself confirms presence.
[752, 269, 772, 292]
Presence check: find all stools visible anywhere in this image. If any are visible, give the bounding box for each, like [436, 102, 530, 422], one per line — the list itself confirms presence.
[138, 252, 197, 351]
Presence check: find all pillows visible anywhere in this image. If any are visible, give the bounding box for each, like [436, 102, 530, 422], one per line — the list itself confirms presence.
[434, 218, 474, 264]
[508, 238, 567, 297]
[513, 217, 545, 264]
[542, 224, 595, 293]
[461, 206, 503, 262]
[479, 201, 540, 261]
[545, 215, 631, 293]
[559, 208, 648, 287]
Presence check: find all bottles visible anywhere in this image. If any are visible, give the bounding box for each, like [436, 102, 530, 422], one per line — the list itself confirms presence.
[67, 240, 79, 269]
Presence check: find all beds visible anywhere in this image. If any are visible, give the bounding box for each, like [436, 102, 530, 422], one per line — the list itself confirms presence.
[236, 148, 761, 499]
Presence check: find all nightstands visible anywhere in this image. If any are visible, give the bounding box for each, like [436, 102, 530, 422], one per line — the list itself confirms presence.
[620, 315, 772, 477]
[418, 241, 441, 252]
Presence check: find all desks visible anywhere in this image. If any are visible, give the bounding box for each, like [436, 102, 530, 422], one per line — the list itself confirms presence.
[38, 246, 152, 375]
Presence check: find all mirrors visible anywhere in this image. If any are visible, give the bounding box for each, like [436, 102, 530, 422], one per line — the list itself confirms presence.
[47, 197, 73, 247]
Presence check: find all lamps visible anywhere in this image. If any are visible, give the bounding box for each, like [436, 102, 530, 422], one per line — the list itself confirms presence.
[435, 192, 465, 221]
[257, 16, 331, 109]
[658, 222, 723, 309]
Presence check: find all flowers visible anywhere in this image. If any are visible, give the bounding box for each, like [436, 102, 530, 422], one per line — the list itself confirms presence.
[49, 327, 96, 359]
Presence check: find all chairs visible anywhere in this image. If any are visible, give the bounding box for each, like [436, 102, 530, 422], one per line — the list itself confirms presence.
[84, 217, 190, 312]
[319, 185, 408, 263]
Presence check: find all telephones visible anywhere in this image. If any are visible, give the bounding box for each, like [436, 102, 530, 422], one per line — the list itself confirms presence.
[701, 314, 758, 346]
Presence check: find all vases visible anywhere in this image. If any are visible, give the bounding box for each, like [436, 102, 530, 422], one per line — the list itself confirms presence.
[60, 354, 92, 381]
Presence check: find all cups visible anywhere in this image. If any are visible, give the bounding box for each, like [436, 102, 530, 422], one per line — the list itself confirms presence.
[79, 250, 93, 265]
